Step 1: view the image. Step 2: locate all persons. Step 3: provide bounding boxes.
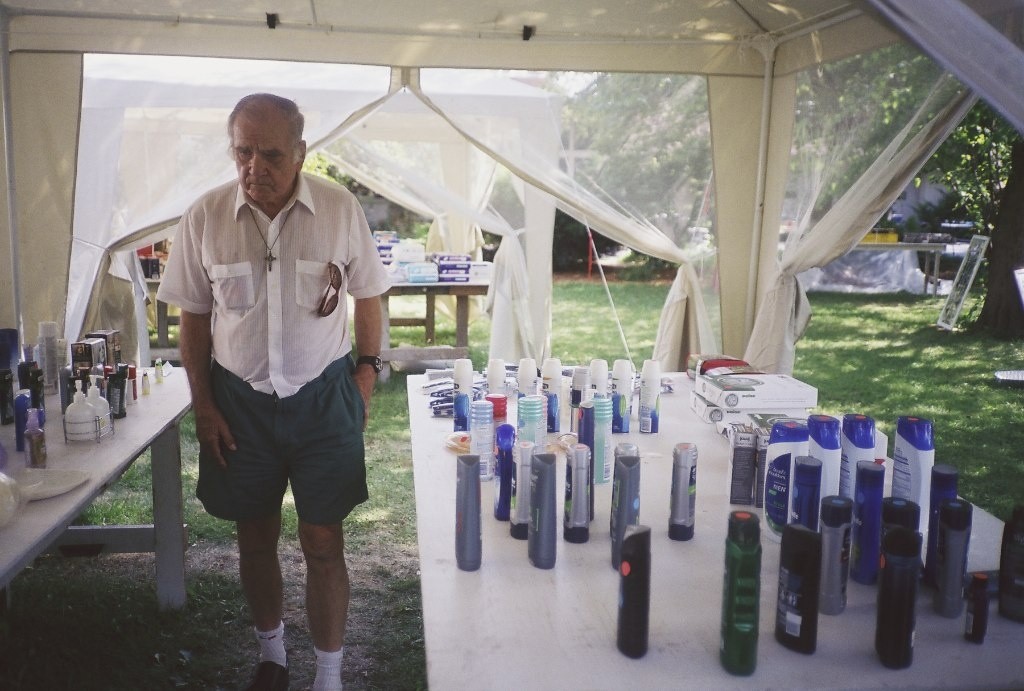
[156,93,393,690]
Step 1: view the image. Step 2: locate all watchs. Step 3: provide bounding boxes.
[357,356,383,373]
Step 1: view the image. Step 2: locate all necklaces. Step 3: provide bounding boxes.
[248,204,292,271]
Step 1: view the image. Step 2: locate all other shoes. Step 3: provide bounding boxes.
[253,655,289,691]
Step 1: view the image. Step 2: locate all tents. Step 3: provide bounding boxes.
[0,0,1024,379]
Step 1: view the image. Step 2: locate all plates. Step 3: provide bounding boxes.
[24,469,92,501]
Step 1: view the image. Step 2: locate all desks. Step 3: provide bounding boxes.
[407,373,1024,691]
[0,366,197,614]
[378,283,489,382]
[852,243,947,297]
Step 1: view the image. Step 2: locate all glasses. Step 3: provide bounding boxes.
[318,262,342,317]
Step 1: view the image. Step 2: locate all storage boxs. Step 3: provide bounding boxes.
[373,230,493,282]
[689,353,819,508]
[60,329,120,415]
